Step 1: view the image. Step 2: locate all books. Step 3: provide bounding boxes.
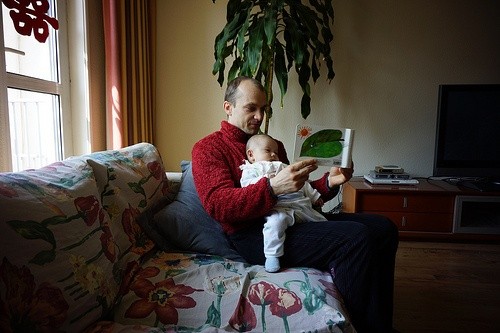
[292,123,356,168]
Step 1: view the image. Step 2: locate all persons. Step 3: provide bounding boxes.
[191,76,400,333]
[239,134,328,272]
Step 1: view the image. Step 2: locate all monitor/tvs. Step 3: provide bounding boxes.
[433,84,500,192]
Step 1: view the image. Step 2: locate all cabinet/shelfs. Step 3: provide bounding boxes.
[342,178,500,241]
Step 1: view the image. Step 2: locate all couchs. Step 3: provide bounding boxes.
[0,142,356,333]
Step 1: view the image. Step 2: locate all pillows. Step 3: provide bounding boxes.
[81,142,177,286]
[0,156,118,333]
